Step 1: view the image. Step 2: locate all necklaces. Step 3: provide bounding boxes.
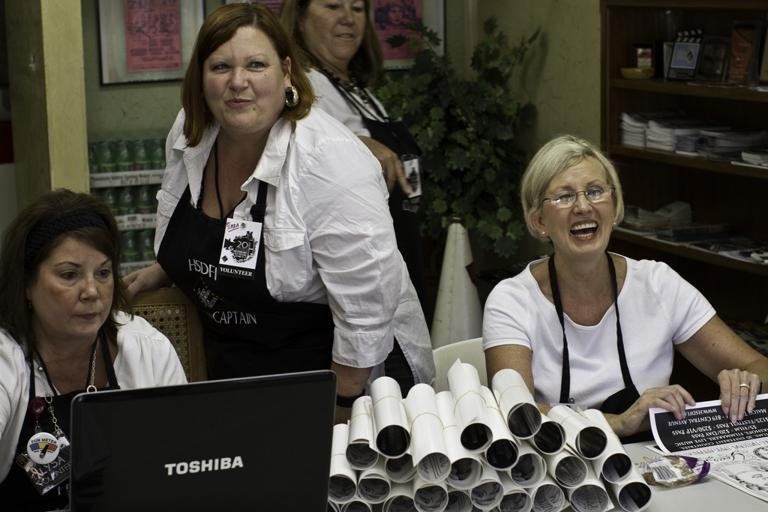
[36,341,96,431]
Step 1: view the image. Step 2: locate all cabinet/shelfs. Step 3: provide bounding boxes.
[598,0,768,277]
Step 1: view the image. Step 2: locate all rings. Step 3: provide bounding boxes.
[740,383,749,388]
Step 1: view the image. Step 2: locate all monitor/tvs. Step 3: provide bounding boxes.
[68,369,338,512]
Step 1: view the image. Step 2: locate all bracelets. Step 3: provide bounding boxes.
[336,389,366,408]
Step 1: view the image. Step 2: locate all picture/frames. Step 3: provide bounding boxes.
[223,0,283,14]
[367,0,447,70]
[96,0,206,88]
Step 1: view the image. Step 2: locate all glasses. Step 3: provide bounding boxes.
[542,183,615,209]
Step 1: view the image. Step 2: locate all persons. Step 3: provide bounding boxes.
[481,133,768,436]
[0,187,188,512]
[119,4,435,425]
[280,0,423,277]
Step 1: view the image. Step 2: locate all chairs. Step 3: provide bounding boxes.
[432,336,488,394]
[115,287,207,384]
